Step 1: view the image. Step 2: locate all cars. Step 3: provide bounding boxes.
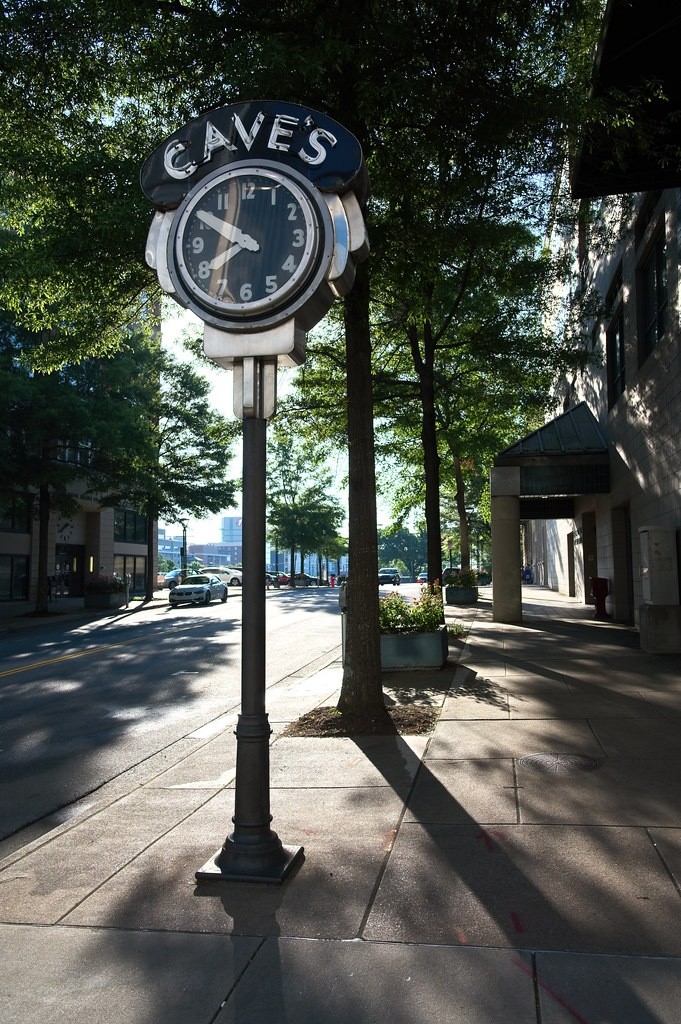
[169,575,228,607]
[441,568,460,584]
[378,568,401,586]
[200,567,242,586]
[415,574,428,583]
[266,571,319,587]
[164,569,203,591]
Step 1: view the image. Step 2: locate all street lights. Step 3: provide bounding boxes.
[179,518,189,582]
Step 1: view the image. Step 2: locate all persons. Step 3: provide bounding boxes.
[521,563,530,584]
